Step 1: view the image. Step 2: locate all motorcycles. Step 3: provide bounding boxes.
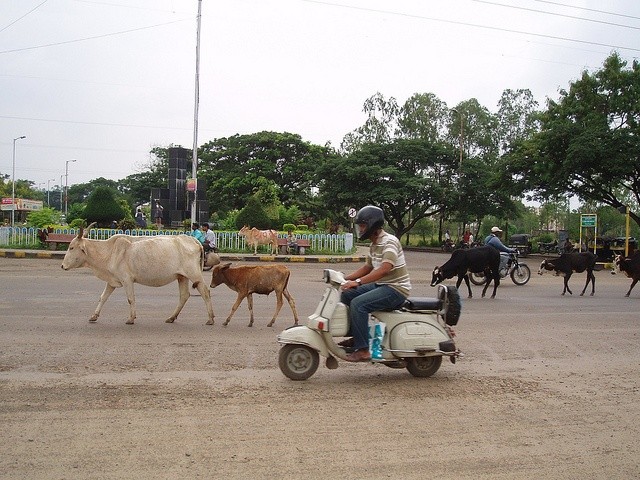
[509,233,532,257]
[469,248,531,285]
[441,238,483,253]
[200,247,219,271]
[275,269,464,381]
[537,239,562,255]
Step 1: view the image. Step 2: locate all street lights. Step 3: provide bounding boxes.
[60,174,68,214]
[47,180,55,208]
[65,160,77,218]
[12,136,26,245]
[39,182,46,193]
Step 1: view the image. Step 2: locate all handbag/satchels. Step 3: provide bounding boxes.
[204,249,220,266]
[367,313,386,359]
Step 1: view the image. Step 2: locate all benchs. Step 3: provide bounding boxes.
[45,233,76,249]
[278,239,310,254]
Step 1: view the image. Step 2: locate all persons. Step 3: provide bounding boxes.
[445,230,449,242]
[338,205,412,361]
[154,200,164,230]
[464,231,469,243]
[468,232,474,244]
[581,241,587,252]
[564,238,571,253]
[286,230,298,255]
[485,226,517,278]
[142,212,147,227]
[137,209,142,225]
[572,240,580,253]
[202,224,216,251]
[190,223,205,245]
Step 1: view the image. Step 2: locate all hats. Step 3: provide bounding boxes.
[491,226,503,233]
[193,223,200,228]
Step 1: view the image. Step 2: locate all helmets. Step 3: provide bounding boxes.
[353,205,384,242]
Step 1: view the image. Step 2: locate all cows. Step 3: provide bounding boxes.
[61,218,214,325]
[210,262,299,327]
[238,222,278,256]
[611,250,640,297]
[430,246,501,299]
[537,251,598,296]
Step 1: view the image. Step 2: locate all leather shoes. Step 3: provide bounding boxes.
[338,338,354,347]
[343,350,371,362]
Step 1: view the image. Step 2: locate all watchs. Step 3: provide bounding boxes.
[355,279,362,285]
